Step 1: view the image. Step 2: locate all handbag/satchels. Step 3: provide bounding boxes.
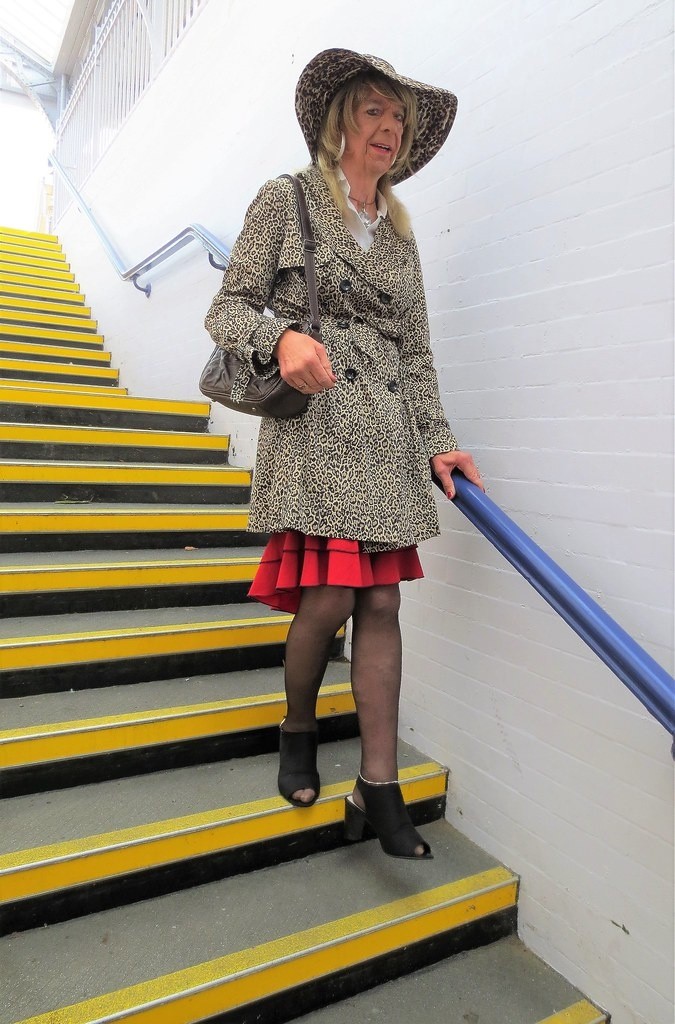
[200,324,321,420]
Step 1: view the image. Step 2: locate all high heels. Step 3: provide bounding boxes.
[342,775,437,863]
[277,718,322,808]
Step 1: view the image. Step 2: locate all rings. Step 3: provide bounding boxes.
[298,383,307,390]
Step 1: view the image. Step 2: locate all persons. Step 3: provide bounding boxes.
[202,45,484,860]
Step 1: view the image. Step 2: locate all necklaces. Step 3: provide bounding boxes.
[348,196,376,229]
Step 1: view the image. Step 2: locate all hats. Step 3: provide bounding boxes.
[297,49,458,186]
